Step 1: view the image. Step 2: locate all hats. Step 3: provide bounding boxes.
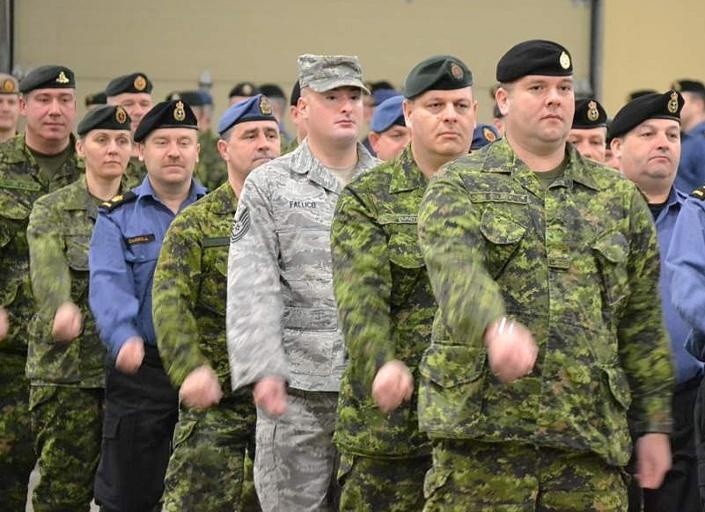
[165,82,284,105]
[366,78,408,134]
[86,72,154,104]
[289,49,371,108]
[76,104,131,135]
[569,99,606,130]
[218,94,277,135]
[133,101,198,141]
[606,89,687,142]
[17,68,75,92]
[494,39,575,84]
[467,108,504,147]
[630,78,702,100]
[403,56,475,98]
[1,73,20,95]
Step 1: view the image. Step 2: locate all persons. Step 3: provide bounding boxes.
[1,39,704,510]
